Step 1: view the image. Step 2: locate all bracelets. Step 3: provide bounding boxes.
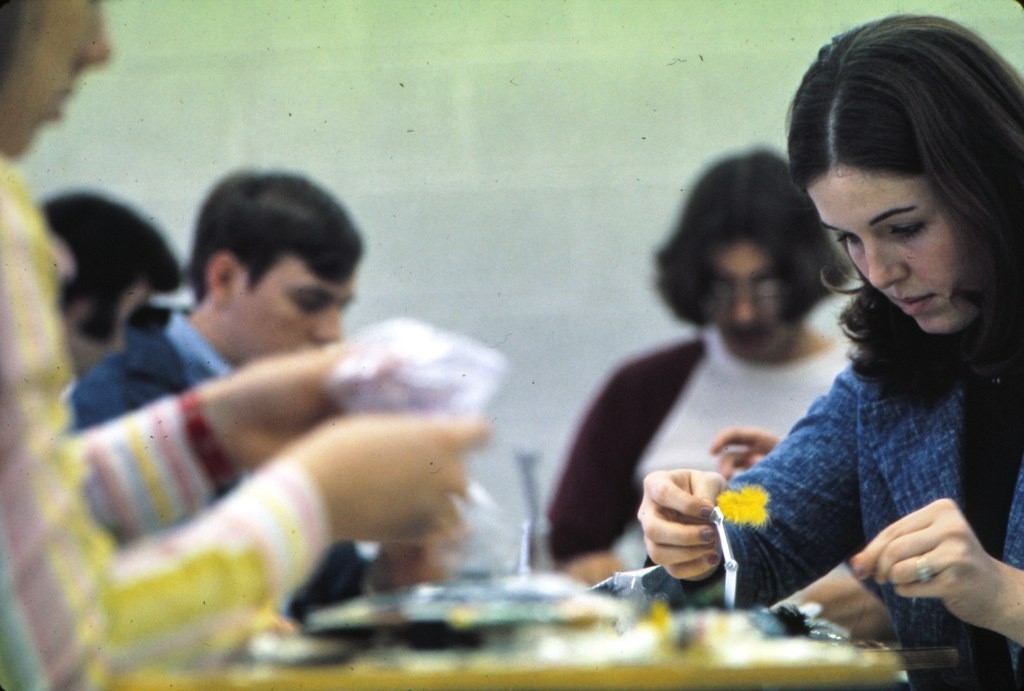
[179,389,239,492]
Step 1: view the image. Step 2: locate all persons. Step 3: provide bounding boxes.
[39,191,182,383]
[547,148,876,599]
[0,0,487,691]
[66,168,500,656]
[636,11,1024,691]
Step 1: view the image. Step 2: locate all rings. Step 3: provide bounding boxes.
[916,555,932,585]
